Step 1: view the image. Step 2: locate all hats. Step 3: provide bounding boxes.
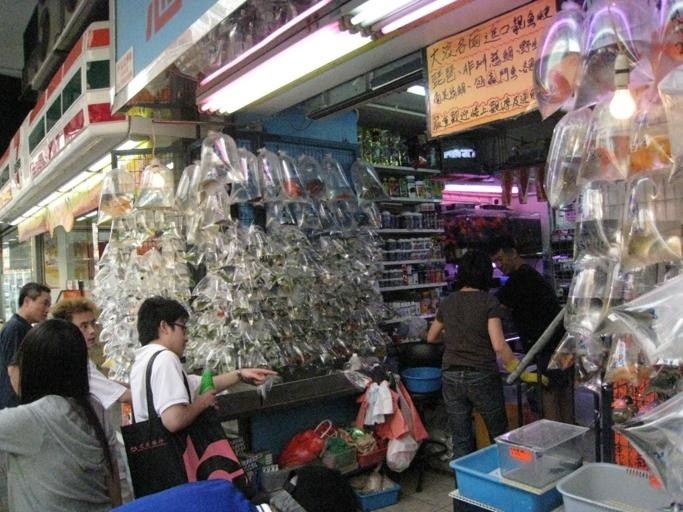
[283,467,357,511]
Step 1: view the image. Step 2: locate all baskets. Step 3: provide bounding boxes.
[322,427,400,510]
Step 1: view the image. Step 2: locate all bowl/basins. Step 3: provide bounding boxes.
[400,367,442,394]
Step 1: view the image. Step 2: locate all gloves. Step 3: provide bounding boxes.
[503,358,549,386]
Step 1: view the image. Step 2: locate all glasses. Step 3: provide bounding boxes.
[168,322,187,332]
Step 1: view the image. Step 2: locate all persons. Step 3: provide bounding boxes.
[482,233,576,425]
[107,465,360,511]
[129,295,279,435]
[48,296,131,410]
[0,319,117,512]
[0,282,52,409]
[427,251,549,493]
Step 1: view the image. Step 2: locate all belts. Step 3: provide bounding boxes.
[447,365,479,372]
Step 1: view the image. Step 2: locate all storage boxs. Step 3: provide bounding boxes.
[493,418,589,489]
[450,486,491,510]
[448,442,590,510]
[555,460,677,511]
[355,472,403,511]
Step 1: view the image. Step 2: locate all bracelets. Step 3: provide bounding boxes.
[236,368,248,384]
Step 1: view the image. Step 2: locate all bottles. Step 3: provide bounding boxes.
[199,369,215,416]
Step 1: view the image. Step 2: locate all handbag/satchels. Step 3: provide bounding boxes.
[277,419,332,465]
[120,403,250,499]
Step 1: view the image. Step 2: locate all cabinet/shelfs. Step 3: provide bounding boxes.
[355,160,447,350]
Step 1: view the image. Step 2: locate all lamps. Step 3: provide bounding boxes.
[606,2,637,120]
[194,0,486,116]
